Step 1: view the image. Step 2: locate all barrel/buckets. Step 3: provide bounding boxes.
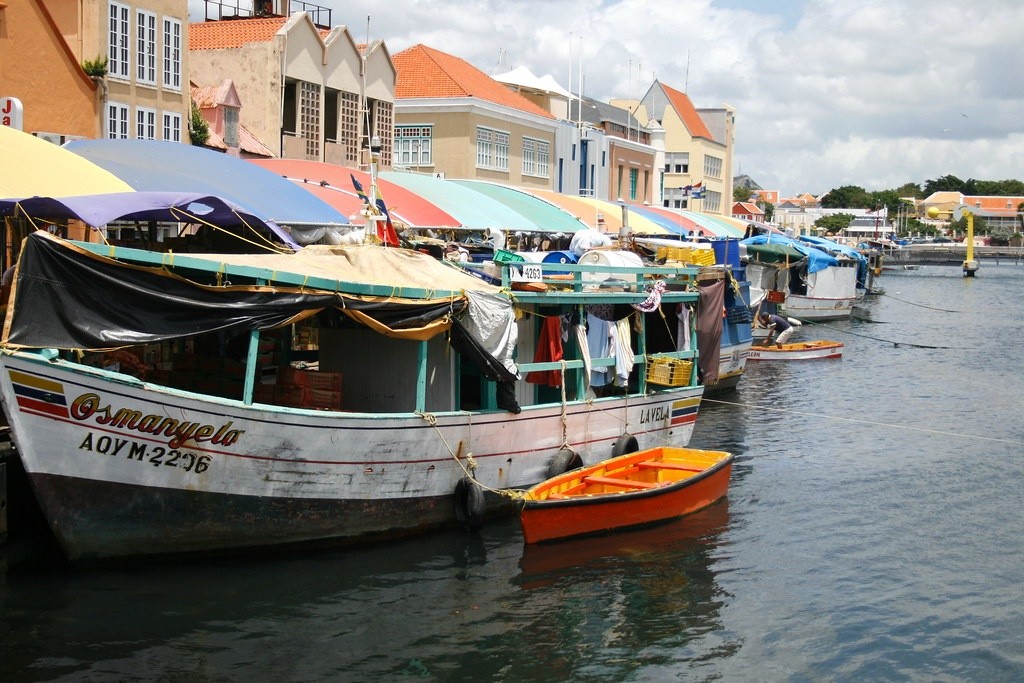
[576,249,646,292]
[542,251,581,279]
[710,239,741,282]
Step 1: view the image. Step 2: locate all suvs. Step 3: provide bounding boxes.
[911,237,927,244]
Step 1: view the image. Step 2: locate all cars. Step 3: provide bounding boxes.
[926,236,935,243]
[933,236,952,243]
[903,237,912,242]
[953,236,964,243]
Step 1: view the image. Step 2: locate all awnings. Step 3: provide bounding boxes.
[244,157,789,241]
[0,124,352,227]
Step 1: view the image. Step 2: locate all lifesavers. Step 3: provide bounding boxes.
[848,242,855,248]
[452,476,487,531]
[612,433,640,458]
[856,243,865,250]
[546,448,585,480]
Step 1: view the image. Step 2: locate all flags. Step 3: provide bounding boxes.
[679,185,692,197]
[349,172,402,248]
[692,187,706,200]
[692,181,702,188]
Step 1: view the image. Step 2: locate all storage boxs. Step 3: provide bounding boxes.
[726,305,754,324]
[274,364,342,411]
[483,249,525,279]
[657,246,716,267]
[81,336,203,394]
[645,356,693,387]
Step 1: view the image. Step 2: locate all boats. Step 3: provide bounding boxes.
[462,233,877,381]
[746,339,843,361]
[0,229,701,567]
[516,493,731,589]
[515,446,736,547]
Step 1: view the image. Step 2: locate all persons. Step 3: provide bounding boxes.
[761,312,795,349]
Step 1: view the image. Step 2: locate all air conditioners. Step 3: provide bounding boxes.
[32,131,61,147]
